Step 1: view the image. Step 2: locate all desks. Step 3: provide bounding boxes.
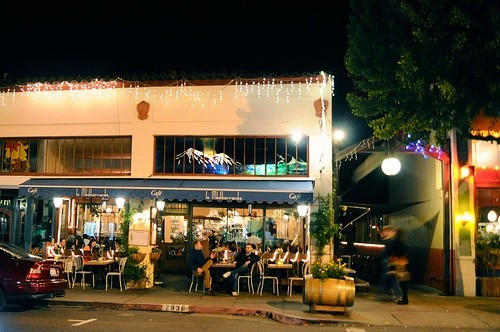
[212,261,237,293]
[268,264,292,295]
[47,254,69,258]
[83,260,115,289]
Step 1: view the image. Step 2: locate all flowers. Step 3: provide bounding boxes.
[276,248,285,255]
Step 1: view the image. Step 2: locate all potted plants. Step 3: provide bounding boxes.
[114,203,162,290]
[473,226,500,297]
[304,193,356,311]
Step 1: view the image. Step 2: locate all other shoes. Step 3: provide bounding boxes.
[223,272,230,278]
[206,289,215,295]
[398,299,409,304]
[232,292,237,296]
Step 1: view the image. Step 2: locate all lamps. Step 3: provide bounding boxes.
[156,201,165,213]
[381,152,401,175]
[487,188,497,223]
[54,198,64,209]
[297,205,307,218]
[116,198,125,208]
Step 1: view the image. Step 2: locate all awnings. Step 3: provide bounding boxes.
[18,179,314,205]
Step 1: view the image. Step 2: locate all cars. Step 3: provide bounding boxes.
[0,242,67,311]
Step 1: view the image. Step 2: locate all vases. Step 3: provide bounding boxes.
[277,255,283,265]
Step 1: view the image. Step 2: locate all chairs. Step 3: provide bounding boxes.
[47,246,127,292]
[189,249,311,298]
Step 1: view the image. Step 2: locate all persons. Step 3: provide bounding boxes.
[382,225,411,305]
[190,240,216,296]
[42,234,122,287]
[268,218,276,236]
[223,244,259,296]
[246,233,261,248]
[209,230,217,250]
[31,243,40,254]
